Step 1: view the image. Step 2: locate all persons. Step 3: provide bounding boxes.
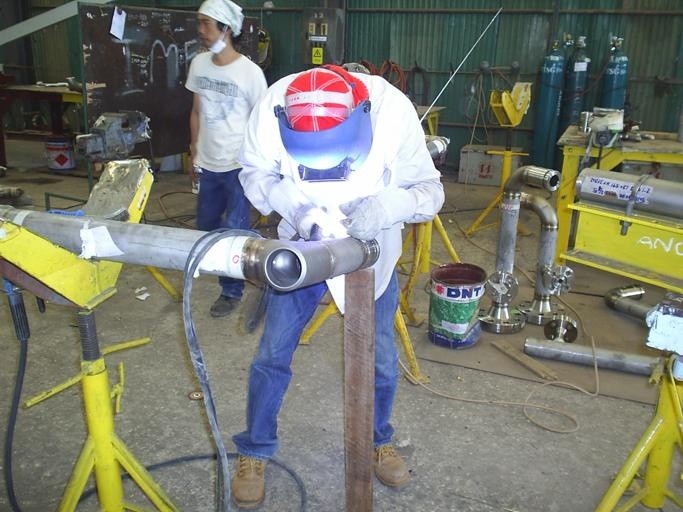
[229,64,446,508]
[184,0,267,316]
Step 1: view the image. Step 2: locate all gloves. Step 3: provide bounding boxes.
[267,175,334,241]
[338,182,417,241]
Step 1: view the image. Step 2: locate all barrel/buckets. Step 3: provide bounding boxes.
[45,139,77,170]
[427,263,490,348]
[182,152,192,173]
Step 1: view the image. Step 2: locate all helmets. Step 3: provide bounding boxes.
[274,63,373,183]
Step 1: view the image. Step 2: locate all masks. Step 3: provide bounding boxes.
[207,25,229,55]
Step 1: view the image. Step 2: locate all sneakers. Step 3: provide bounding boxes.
[209,295,242,317]
[230,454,266,508]
[373,443,411,487]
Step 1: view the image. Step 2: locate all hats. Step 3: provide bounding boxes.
[197,0,245,39]
